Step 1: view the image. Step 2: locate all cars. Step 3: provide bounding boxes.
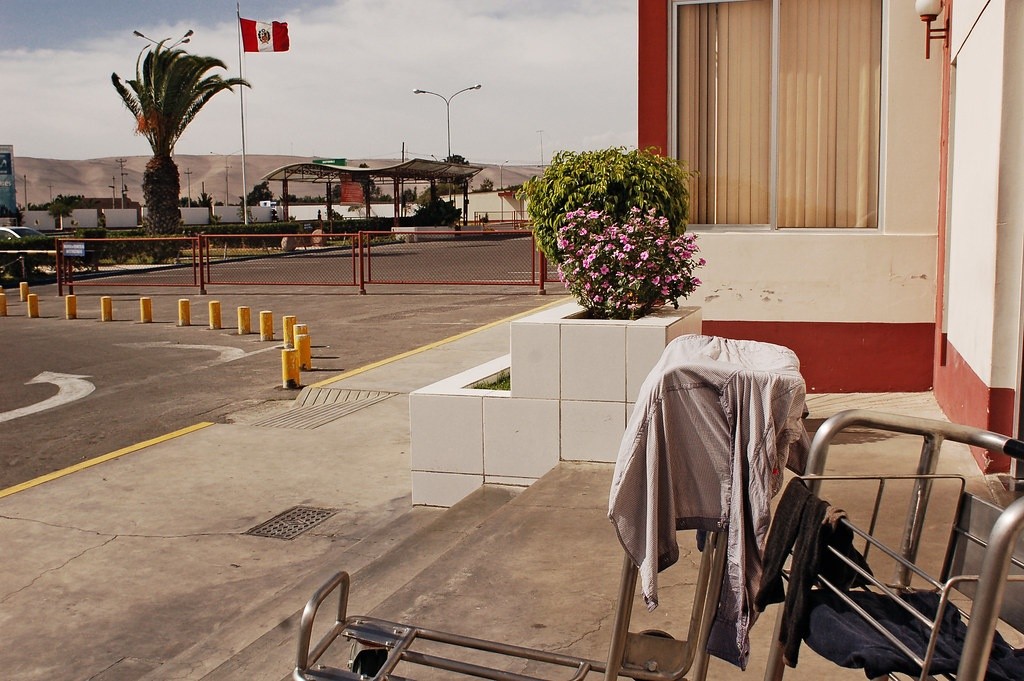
[0,226,46,241]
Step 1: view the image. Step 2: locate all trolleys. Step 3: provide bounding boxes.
[295,334,811,681]
[757,411,1024,681]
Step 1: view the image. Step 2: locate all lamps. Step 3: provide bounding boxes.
[915,0,950,60]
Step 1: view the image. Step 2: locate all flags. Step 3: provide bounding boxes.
[240,17,289,53]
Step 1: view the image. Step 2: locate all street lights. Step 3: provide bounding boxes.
[133,29,193,159]
[210,149,242,206]
[493,160,509,221]
[413,84,482,204]
